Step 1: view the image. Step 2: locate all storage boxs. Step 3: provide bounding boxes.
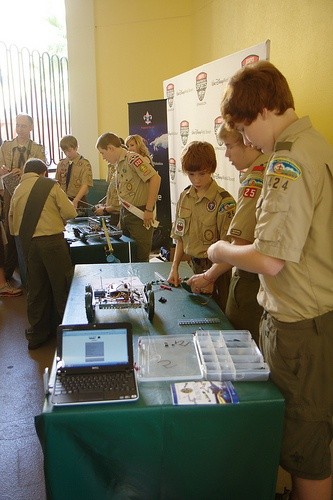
[135,328,271,383]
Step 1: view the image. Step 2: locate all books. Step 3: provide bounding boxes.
[171,379,241,406]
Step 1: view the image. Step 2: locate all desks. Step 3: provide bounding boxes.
[34,261,285,500]
[60,214,138,266]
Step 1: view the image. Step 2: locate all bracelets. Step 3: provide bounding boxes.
[102,208,109,216]
[145,207,154,212]
[203,270,215,284]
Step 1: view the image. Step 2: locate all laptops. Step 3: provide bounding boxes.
[50,323,139,406]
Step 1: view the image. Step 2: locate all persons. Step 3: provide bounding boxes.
[126,132,154,161]
[209,56,333,500]
[56,136,92,217]
[95,132,162,263]
[184,118,263,348]
[0,191,23,296]
[166,141,236,312]
[10,158,78,350]
[92,138,126,228]
[0,114,49,281]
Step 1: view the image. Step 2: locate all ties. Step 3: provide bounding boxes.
[17,147,26,168]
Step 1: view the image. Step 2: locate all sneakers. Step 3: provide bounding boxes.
[0,281,23,296]
